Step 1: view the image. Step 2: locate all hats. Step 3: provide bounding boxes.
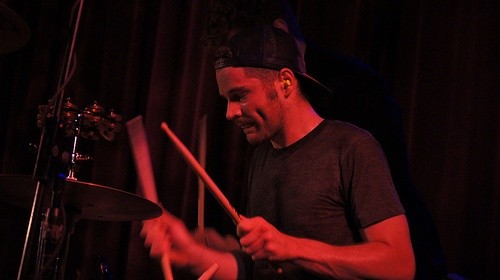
[213,23,322,88]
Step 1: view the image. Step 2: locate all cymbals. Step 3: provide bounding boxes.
[0,174,162,221]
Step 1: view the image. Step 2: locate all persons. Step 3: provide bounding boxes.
[139,24,417,280]
